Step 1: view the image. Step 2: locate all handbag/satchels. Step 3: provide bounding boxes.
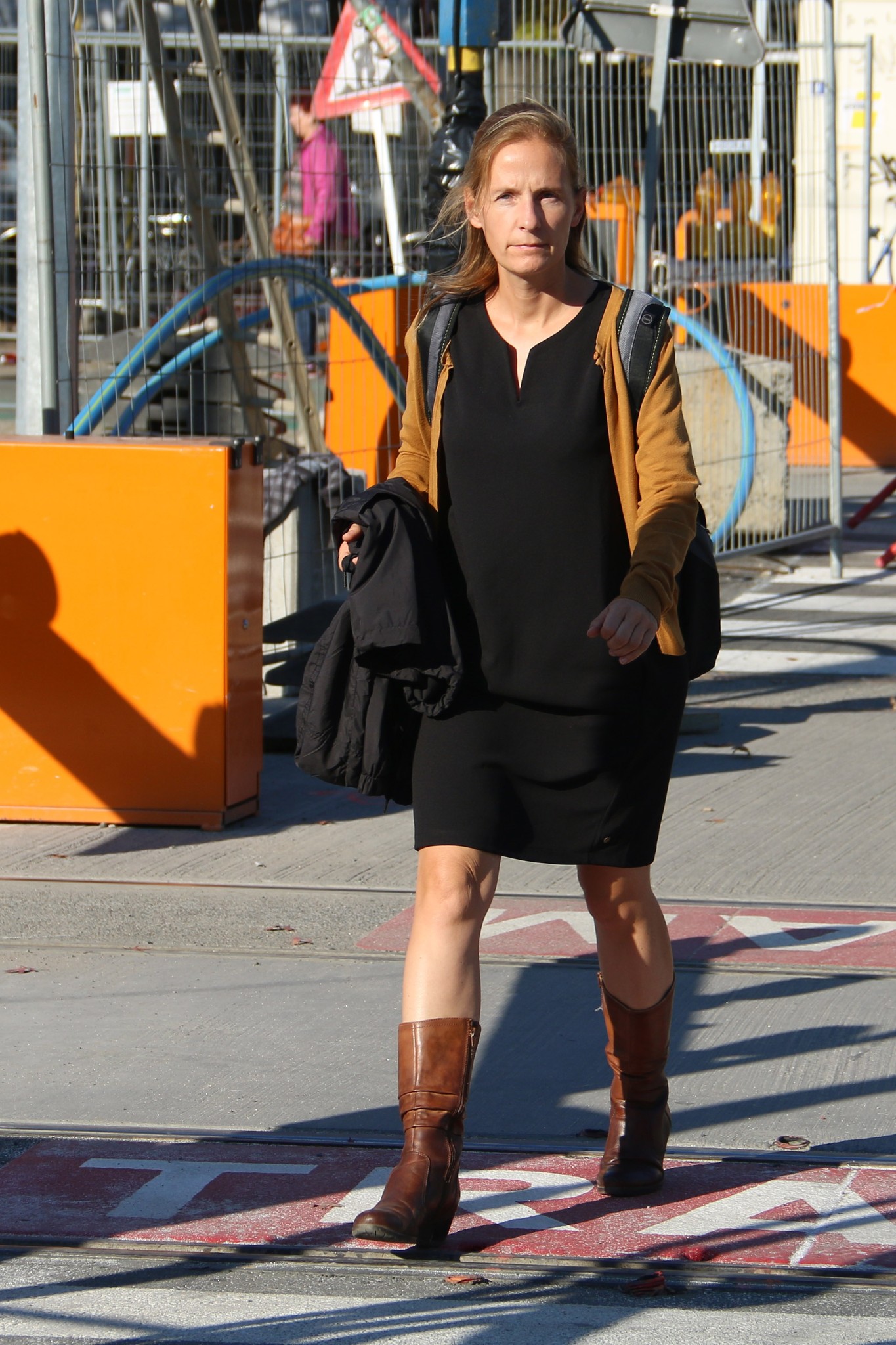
[618,290,723,679]
[272,214,316,257]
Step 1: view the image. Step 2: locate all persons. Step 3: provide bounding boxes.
[337,103,700,1242]
[277,95,357,374]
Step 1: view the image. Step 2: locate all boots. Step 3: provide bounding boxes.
[352,1017,482,1244]
[594,971,676,1196]
